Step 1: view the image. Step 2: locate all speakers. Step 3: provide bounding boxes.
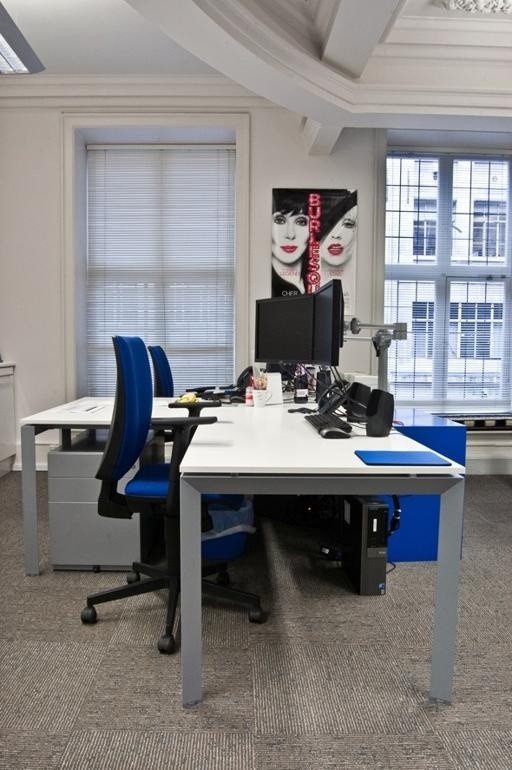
[238,366,253,387]
[365,388,395,437]
[346,382,371,423]
[316,371,331,401]
[295,375,308,403]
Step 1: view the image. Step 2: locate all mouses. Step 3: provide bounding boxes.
[320,426,351,439]
[230,395,245,403]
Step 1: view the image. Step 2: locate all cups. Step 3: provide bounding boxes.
[252,389,271,407]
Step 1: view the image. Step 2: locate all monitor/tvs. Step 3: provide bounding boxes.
[312,279,344,367]
[255,293,314,364]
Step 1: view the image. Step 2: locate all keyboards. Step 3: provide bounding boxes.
[305,412,352,433]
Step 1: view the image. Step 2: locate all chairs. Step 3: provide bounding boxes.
[81,339,268,656]
[148,344,221,444]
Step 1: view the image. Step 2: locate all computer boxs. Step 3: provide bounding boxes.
[339,495,389,597]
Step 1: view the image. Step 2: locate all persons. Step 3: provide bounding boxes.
[272,189,307,296]
[314,189,358,291]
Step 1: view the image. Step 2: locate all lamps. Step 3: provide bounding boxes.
[0,4,45,75]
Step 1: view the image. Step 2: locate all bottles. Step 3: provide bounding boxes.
[294,370,308,404]
[245,386,255,408]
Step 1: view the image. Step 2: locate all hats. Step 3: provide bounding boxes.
[316,189,357,241]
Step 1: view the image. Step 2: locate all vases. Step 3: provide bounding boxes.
[253,390,269,407]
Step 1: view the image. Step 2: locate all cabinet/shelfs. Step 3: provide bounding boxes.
[47,430,166,570]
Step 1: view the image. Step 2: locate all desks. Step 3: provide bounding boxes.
[23,394,467,706]
[387,407,468,564]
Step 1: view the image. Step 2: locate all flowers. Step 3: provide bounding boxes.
[250,374,270,390]
